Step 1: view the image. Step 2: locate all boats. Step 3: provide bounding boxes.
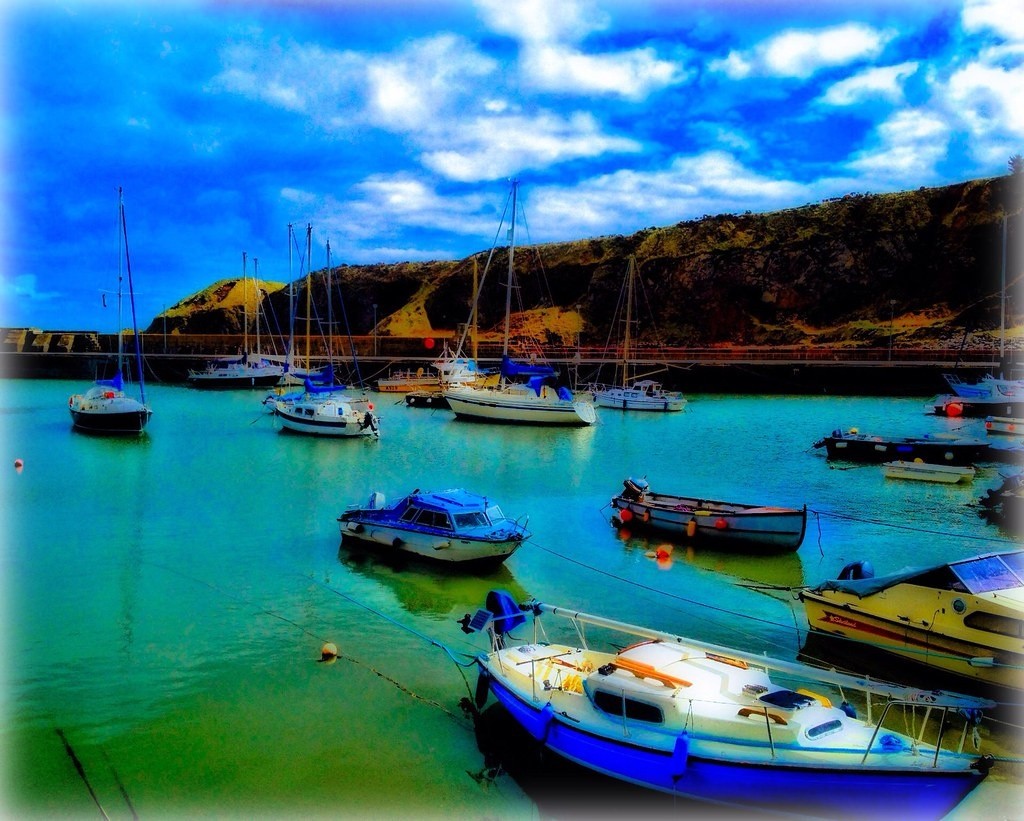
[977,473,1023,518]
[616,531,804,608]
[923,393,1023,417]
[796,548,1023,735]
[336,488,534,574]
[611,477,807,555]
[813,428,993,466]
[984,415,1024,436]
[405,386,451,409]
[457,590,999,821]
[881,460,975,484]
[337,548,535,621]
[376,368,442,392]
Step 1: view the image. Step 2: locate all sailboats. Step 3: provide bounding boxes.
[435,177,691,426]
[186,220,382,439]
[67,186,154,438]
[942,207,1023,398]
